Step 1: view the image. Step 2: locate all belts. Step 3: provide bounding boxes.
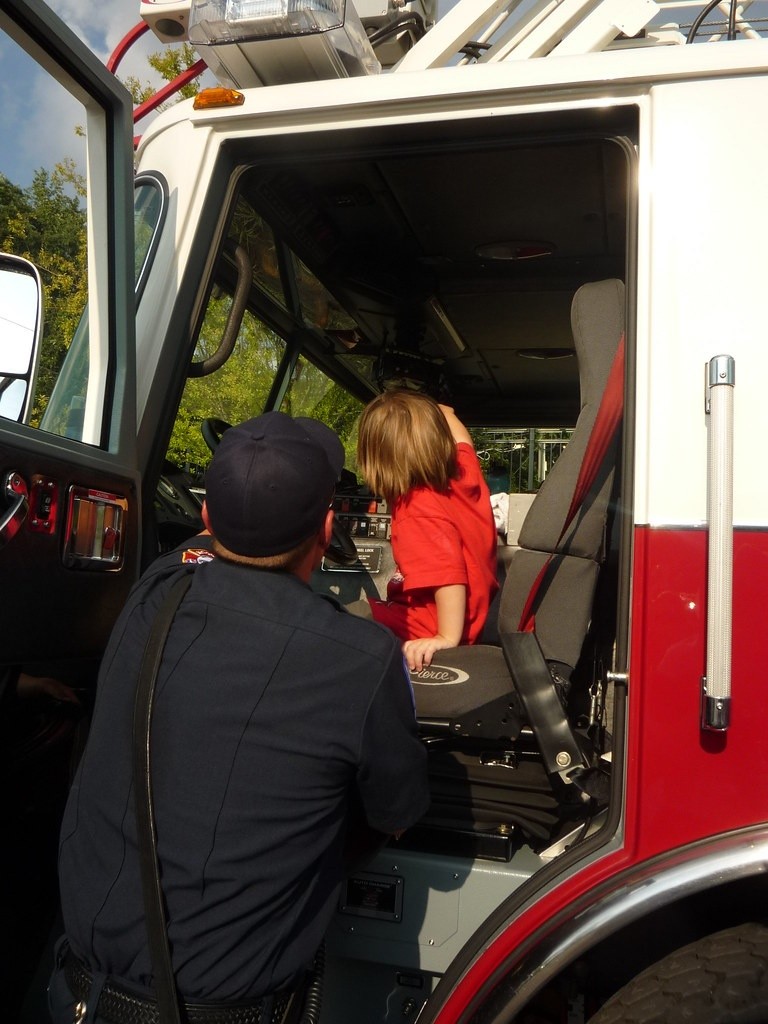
[65,960,288,1024]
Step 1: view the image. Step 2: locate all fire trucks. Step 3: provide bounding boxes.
[0,0,767,1022]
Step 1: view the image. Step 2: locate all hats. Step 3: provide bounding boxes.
[206,411,345,558]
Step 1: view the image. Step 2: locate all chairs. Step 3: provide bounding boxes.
[401,278,628,725]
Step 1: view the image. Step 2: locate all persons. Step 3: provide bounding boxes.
[356,389,498,673]
[44,410,431,1024]
